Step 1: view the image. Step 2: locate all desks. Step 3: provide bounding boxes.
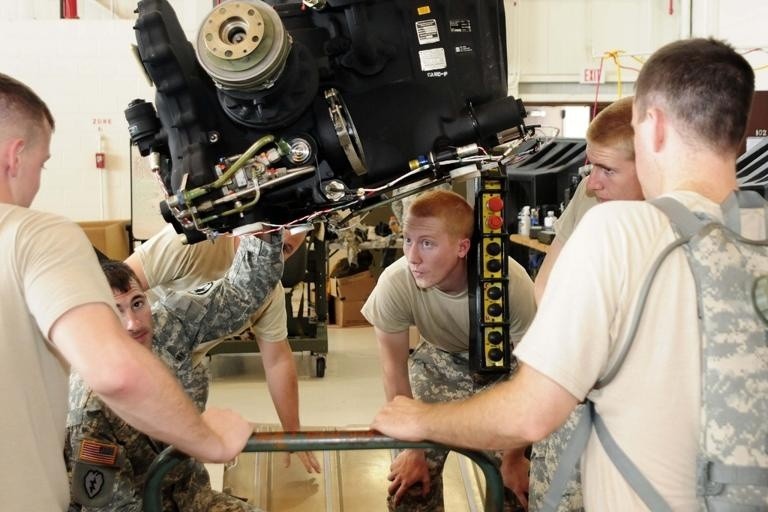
[508,233,551,264]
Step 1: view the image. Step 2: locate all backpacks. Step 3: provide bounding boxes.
[542,189,764,510]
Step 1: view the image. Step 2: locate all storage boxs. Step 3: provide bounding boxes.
[329,273,378,301]
[74,218,133,267]
[333,297,376,331]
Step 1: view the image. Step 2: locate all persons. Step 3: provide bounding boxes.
[389,179,454,228]
[119,216,322,474]
[58,224,286,511]
[357,189,540,511]
[370,35,768,512]
[528,94,647,512]
[0,71,257,511]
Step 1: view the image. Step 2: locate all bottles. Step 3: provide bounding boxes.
[544,211,557,232]
[388,216,399,234]
[517,205,539,237]
